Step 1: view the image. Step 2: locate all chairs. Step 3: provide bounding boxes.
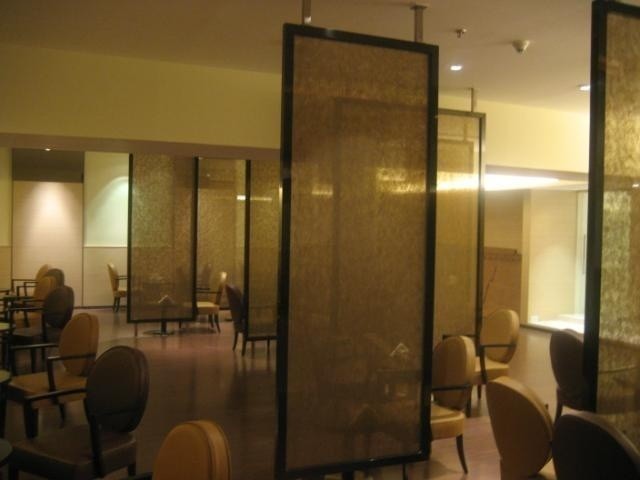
[550,329,639,480]
[107,263,127,313]
[178,263,228,335]
[442,310,520,418]
[486,376,555,480]
[134,323,137,337]
[365,335,476,480]
[8,345,150,480]
[1,263,100,480]
[224,283,276,356]
[118,419,232,480]
